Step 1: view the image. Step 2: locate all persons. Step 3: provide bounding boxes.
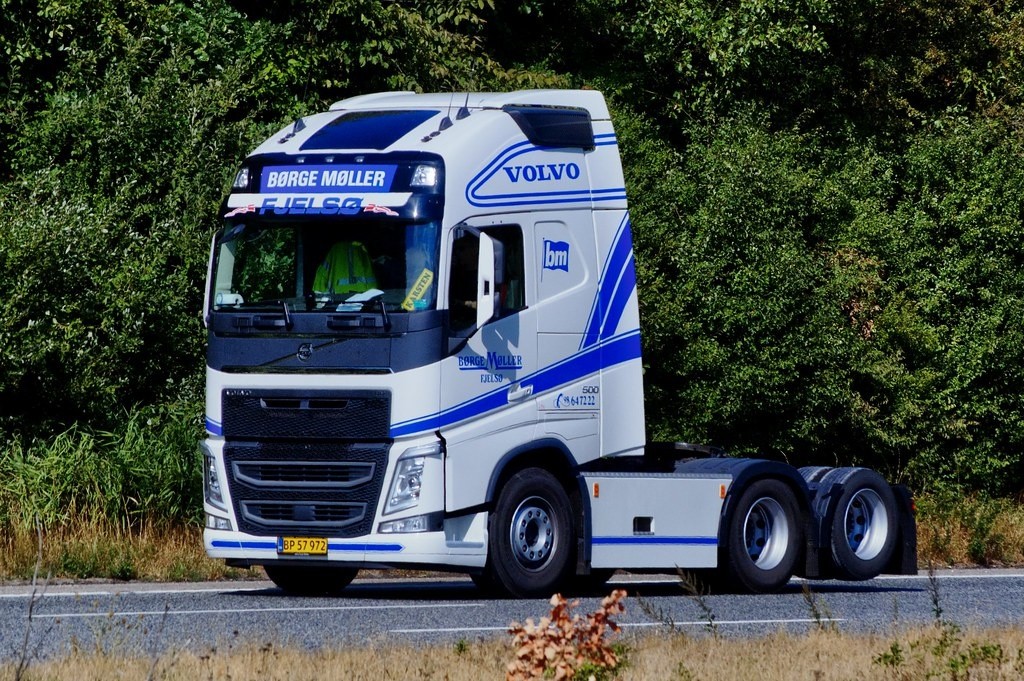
[451,235,509,332]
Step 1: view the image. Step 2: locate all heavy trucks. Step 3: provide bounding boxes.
[197,86,921,598]
